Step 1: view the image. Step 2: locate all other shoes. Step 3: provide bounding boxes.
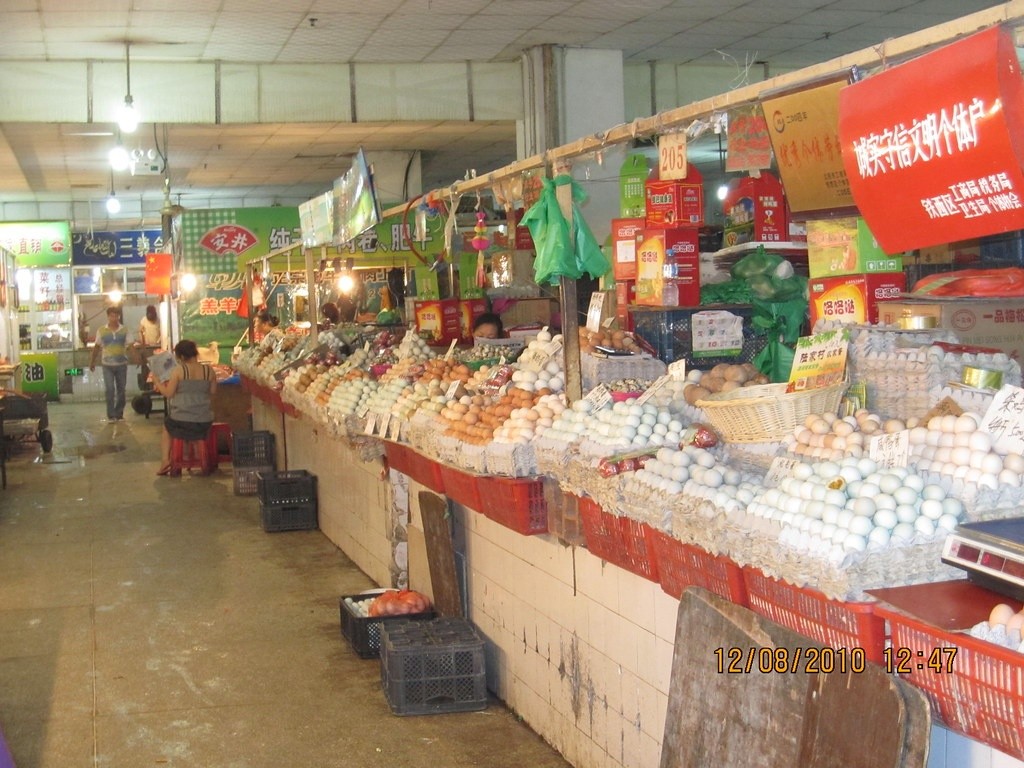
[117,416,124,422]
[108,417,115,423]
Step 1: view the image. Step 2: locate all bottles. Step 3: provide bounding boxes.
[17,298,72,312]
[663,249,679,306]
[20,320,73,351]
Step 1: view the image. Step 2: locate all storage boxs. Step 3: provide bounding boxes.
[233,465,278,497]
[5,395,48,431]
[231,429,276,468]
[581,155,1024,386]
[255,470,321,529]
[3,417,40,437]
[403,206,551,341]
[338,591,489,717]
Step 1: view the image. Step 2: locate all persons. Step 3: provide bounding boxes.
[471,312,503,349]
[257,313,283,351]
[92,307,135,424]
[140,305,162,392]
[151,340,217,476]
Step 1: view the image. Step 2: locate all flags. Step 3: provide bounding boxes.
[145,254,172,295]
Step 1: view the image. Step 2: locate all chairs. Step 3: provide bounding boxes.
[139,353,168,420]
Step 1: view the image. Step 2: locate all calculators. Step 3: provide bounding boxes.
[595,344,635,355]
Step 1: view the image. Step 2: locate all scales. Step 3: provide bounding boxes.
[939,515,1024,604]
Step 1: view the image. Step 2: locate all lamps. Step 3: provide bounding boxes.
[104,41,140,217]
[109,281,123,305]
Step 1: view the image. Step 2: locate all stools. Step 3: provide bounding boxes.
[169,419,235,481]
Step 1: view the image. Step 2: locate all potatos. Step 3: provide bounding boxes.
[682,362,769,406]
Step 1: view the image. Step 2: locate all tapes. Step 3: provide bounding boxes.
[960,365,1002,390]
[897,315,937,328]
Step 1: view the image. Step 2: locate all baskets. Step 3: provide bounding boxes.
[694,381,852,442]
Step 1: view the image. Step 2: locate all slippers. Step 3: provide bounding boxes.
[157,464,172,475]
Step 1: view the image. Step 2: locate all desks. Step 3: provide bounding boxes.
[0,362,23,394]
[209,383,252,454]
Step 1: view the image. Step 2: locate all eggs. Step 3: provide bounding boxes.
[927,345,943,361]
[345,590,430,617]
[258,330,688,464]
[989,604,1024,641]
[631,409,1024,551]
